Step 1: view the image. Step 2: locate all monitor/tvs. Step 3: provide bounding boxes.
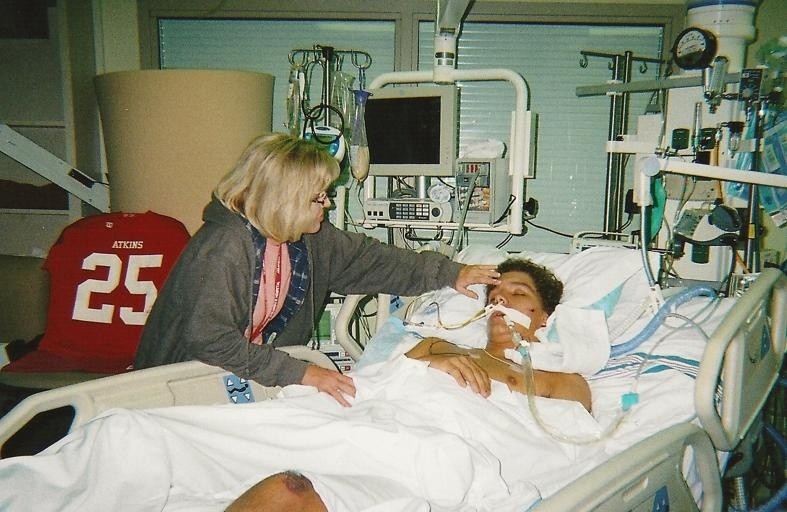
[362,89,460,178]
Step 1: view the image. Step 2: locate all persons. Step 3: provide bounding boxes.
[219,257,591,511]
[131,135,501,409]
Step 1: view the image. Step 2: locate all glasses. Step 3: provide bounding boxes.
[308,190,338,208]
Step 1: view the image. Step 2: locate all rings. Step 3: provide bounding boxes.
[335,388,341,391]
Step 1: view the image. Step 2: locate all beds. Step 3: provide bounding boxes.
[0,267,787,512]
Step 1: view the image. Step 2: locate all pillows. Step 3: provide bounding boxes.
[407,243,659,346]
